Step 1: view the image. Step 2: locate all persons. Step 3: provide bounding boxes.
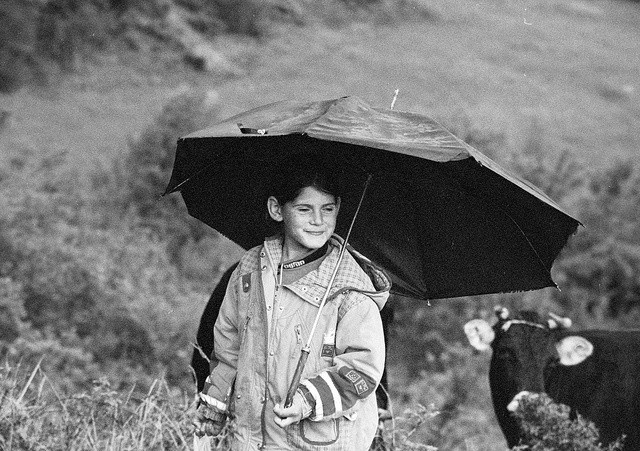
[191,157,392,450]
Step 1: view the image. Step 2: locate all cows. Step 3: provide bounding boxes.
[462,304,640,451]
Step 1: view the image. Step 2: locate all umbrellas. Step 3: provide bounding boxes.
[162,86,585,419]
